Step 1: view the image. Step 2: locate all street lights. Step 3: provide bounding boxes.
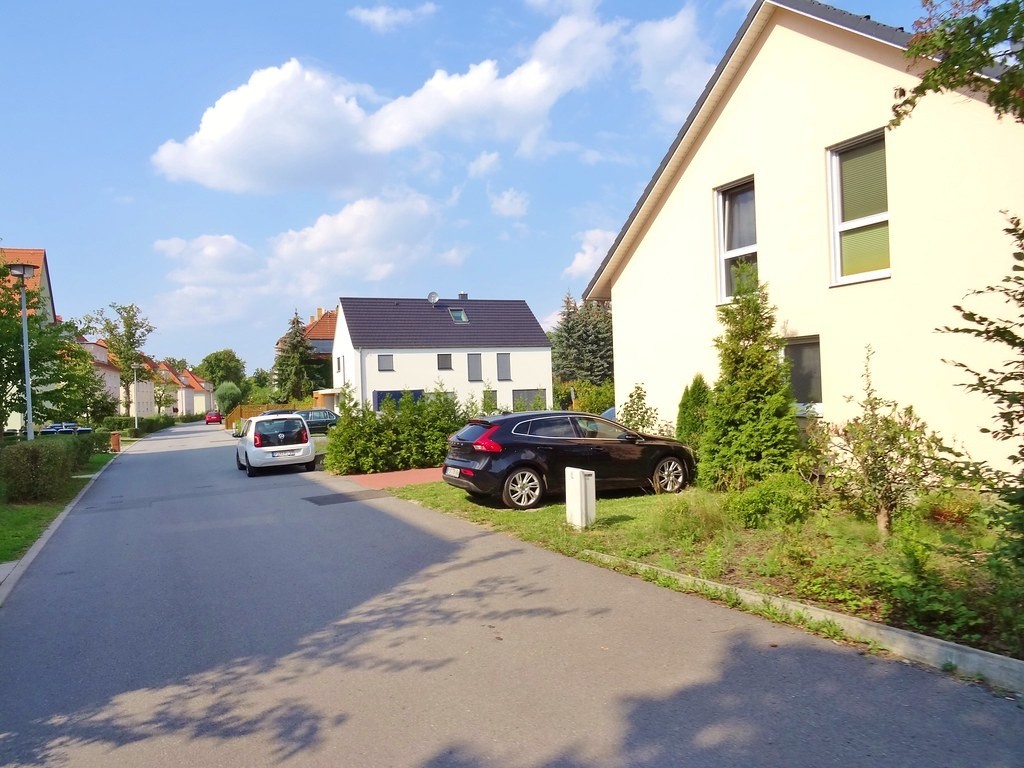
[4,262,40,443]
[130,362,141,428]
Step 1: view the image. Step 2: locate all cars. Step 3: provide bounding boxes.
[231,413,316,477]
[442,409,697,511]
[205,412,224,424]
[258,408,299,431]
[293,409,341,435]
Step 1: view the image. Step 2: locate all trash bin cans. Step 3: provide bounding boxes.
[110,431,122,452]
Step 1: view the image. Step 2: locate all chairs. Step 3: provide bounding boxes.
[257,423,270,434]
[284,421,297,431]
